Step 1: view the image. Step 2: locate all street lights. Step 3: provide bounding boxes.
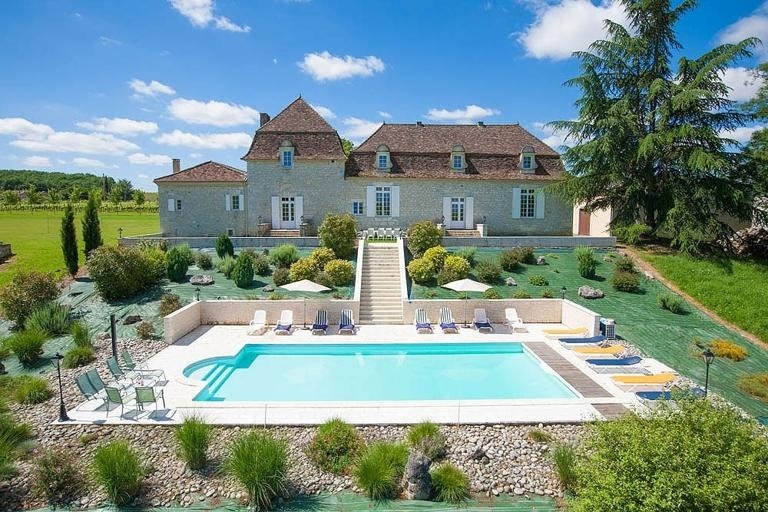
[195,287,201,300]
[561,286,566,298]
[49,351,70,422]
[701,348,714,400]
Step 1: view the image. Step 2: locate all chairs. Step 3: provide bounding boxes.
[73,351,167,420]
[415,307,526,334]
[246,309,354,335]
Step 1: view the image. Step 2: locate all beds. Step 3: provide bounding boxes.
[542,327,705,403]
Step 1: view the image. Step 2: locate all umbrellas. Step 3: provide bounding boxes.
[441,278,494,321]
[278,278,332,323]
